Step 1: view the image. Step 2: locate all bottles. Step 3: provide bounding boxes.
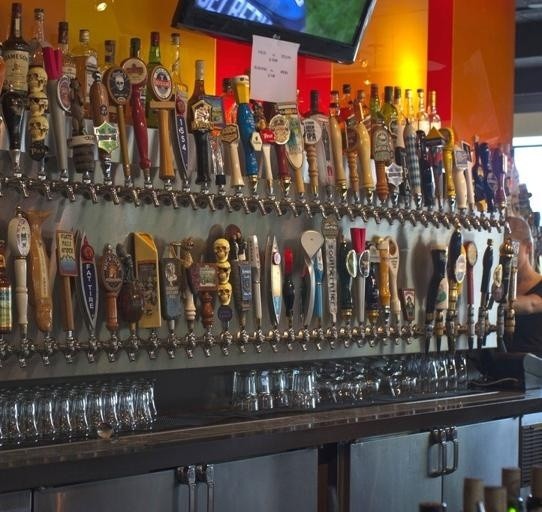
[0,0,442,168]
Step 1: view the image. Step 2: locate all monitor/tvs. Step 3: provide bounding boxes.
[171,0,377,65]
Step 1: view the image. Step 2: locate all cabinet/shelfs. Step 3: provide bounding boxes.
[349,419,523,512]
[33,446,320,512]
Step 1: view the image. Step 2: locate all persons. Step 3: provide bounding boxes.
[497,214,542,361]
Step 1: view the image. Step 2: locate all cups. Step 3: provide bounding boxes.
[231,353,469,414]
[0,377,158,448]
